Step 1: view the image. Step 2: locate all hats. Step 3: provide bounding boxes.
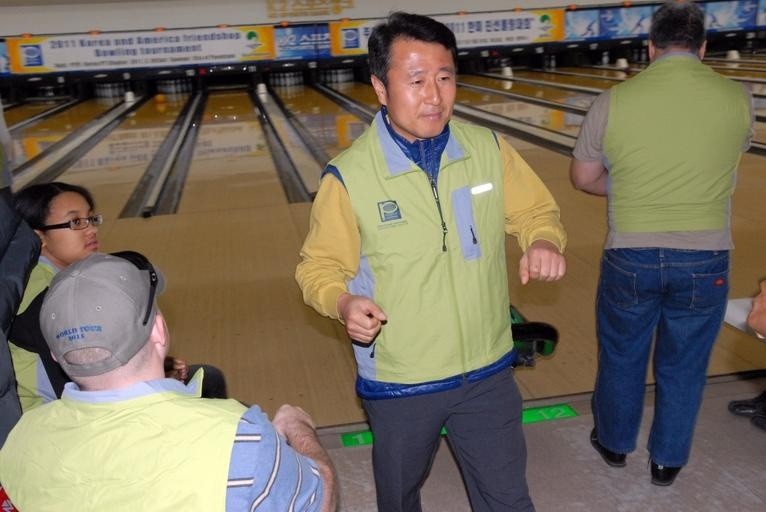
[38,251,165,378]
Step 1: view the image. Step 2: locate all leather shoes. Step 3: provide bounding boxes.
[751,413,766,430]
[650,456,681,486]
[590,427,626,466]
[729,395,765,416]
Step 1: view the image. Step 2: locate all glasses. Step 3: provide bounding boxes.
[37,213,102,230]
[109,251,158,326]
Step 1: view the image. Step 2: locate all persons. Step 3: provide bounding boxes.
[294,11,568,512]
[0,251,338,512]
[570,1,756,486]
[8,183,227,414]
[728,278,766,431]
